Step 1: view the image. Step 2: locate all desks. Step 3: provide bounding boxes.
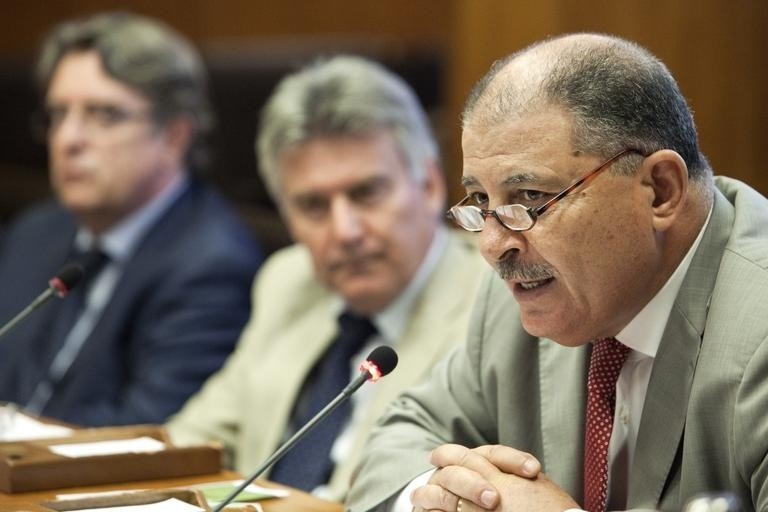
[1,412,342,512]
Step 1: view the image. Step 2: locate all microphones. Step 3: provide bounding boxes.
[212,345,399,512]
[0,263,83,337]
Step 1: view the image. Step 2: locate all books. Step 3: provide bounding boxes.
[0,424,224,496]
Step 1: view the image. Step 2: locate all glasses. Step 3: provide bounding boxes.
[448,148,649,232]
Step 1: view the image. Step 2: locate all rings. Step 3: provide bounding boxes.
[455,496,463,512]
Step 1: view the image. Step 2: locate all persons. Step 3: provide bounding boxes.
[154,50,500,512]
[338,29,768,512]
[0,9,264,431]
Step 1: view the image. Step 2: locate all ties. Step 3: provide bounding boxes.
[583,336,633,512]
[12,245,110,403]
[264,313,379,493]
